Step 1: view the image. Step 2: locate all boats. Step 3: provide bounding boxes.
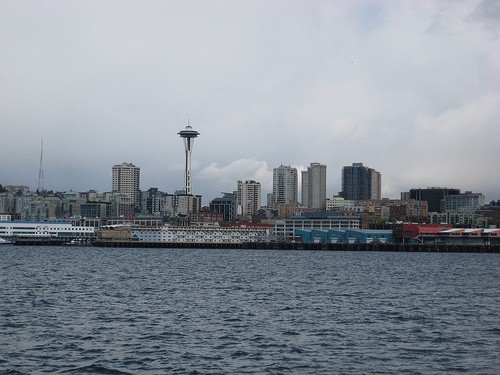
[0,219,97,245]
